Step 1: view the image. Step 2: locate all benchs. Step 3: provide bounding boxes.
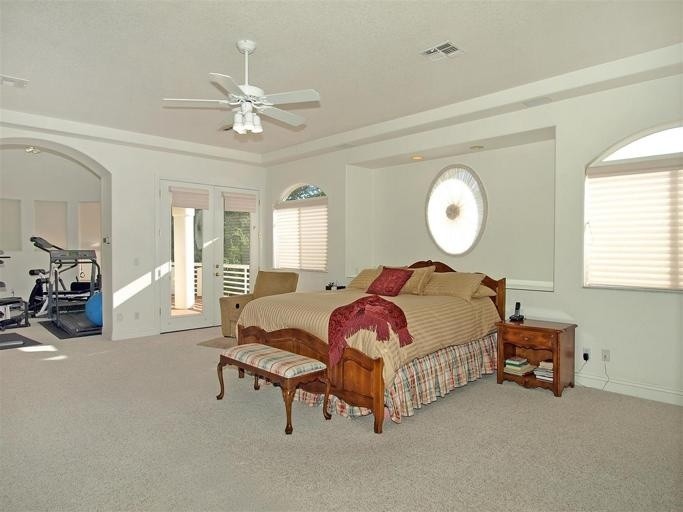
[216,342,332,434]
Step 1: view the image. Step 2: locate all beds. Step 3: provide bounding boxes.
[235,260,506,433]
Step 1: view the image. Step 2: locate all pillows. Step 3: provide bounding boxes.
[366,267,415,297]
[364,265,436,295]
[472,283,498,299]
[424,272,487,303]
[347,269,377,290]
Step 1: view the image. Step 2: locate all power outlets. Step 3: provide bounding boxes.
[602,349,610,362]
[583,347,592,359]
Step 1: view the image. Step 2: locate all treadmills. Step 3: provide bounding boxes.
[49,250,102,337]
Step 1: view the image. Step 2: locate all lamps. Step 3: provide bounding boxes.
[25,145,40,154]
[232,101,263,134]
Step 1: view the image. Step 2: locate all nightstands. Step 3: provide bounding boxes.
[495,319,577,397]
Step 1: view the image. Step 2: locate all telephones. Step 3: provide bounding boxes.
[509,302,524,321]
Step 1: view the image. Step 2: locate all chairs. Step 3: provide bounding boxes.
[219,271,299,338]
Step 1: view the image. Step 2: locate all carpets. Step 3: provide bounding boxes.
[197,337,238,349]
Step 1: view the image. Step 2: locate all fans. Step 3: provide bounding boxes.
[162,40,321,128]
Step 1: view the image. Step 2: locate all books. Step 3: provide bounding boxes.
[501,355,553,384]
